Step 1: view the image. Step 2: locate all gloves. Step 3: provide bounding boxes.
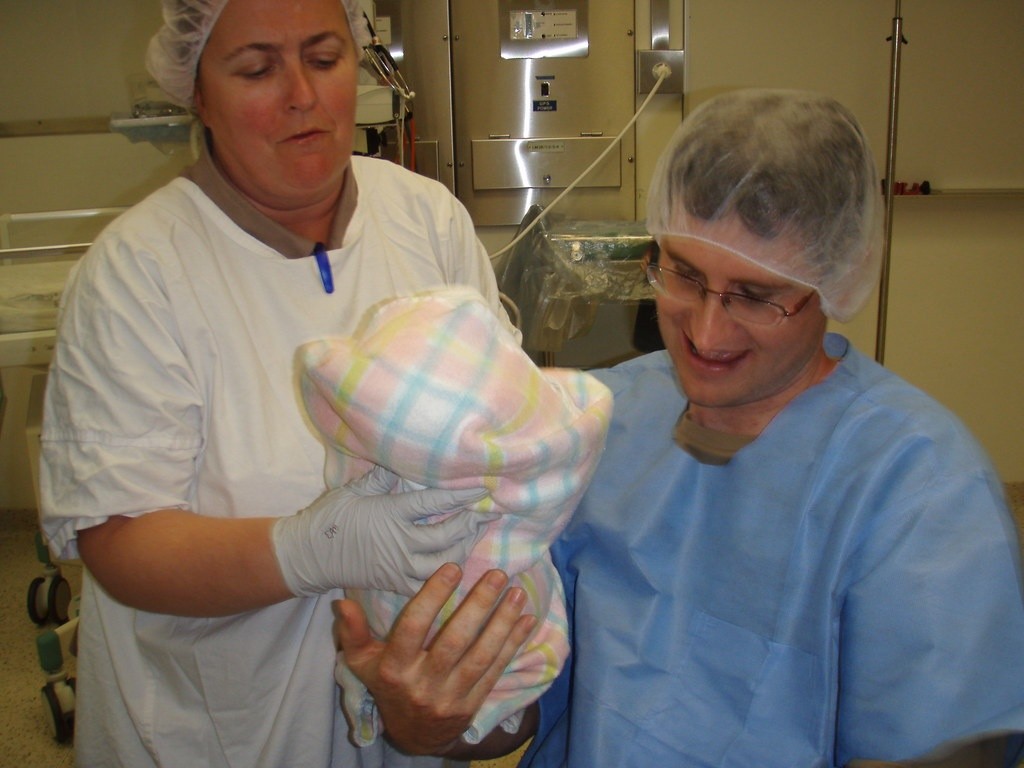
[272,465,505,602]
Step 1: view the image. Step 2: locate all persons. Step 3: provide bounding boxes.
[294,283,612,749]
[32,1,521,768]
[331,90,1024,768]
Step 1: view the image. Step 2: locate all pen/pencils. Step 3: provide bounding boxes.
[308,241,339,294]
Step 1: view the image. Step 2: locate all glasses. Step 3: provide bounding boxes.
[645,262,819,327]
